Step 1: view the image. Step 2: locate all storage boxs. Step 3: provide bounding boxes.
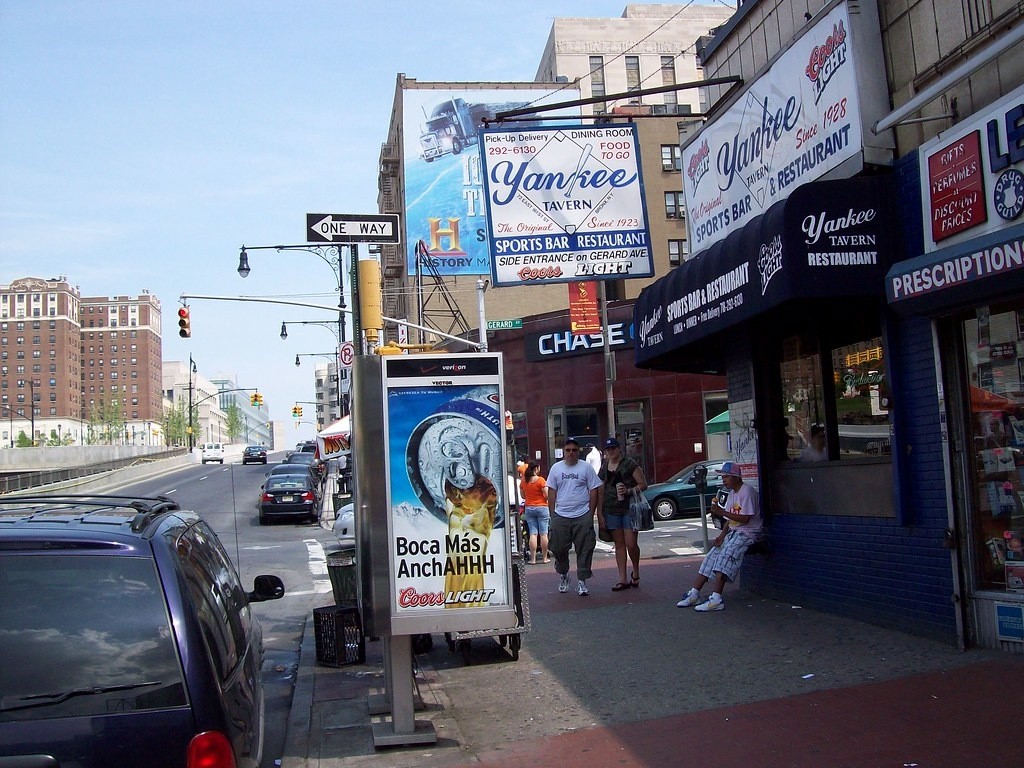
[314,603,366,668]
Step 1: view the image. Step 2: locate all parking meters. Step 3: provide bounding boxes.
[693,464,711,555]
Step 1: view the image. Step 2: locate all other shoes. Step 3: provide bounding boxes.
[543,559,551,563]
[527,560,536,565]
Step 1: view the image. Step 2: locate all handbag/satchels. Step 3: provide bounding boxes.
[629,487,655,532]
[598,519,614,542]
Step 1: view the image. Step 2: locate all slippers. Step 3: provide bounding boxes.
[612,582,631,591]
[631,571,640,587]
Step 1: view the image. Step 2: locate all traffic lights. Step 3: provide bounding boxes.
[250,393,263,406]
[291,405,302,417]
[177,306,191,337]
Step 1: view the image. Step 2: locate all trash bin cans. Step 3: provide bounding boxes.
[332,490,353,519]
[327,547,359,627]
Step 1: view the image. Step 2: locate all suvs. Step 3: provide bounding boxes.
[1,495,285,768]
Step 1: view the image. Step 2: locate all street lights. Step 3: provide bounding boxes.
[106,416,180,446]
[5,377,34,448]
[58,424,61,447]
[188,352,197,453]
[280,320,350,417]
[87,424,90,446]
[236,242,364,358]
[205,416,249,444]
[294,353,344,418]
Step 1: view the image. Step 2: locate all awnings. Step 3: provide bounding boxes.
[705,410,731,435]
[632,180,905,379]
[314,414,351,462]
[884,221,1024,320]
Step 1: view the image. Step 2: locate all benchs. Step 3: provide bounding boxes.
[274,483,303,488]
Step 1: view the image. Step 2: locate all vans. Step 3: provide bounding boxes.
[200,442,224,464]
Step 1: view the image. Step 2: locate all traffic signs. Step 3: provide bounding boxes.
[305,212,400,247]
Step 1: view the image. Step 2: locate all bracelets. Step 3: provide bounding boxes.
[720,509,725,515]
[628,489,631,496]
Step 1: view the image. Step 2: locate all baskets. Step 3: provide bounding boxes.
[313,606,366,666]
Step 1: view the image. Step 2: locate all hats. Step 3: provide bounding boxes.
[565,437,579,445]
[583,443,595,449]
[714,462,742,477]
[811,425,826,437]
[604,438,621,449]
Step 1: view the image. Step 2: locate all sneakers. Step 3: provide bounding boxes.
[577,579,590,596]
[694,595,725,611]
[677,591,701,607]
[559,573,572,593]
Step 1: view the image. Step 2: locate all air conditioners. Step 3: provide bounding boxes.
[681,211,685,219]
[663,164,674,171]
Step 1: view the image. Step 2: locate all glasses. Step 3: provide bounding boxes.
[564,447,578,453]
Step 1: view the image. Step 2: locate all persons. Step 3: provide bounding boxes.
[987,419,1007,449]
[676,462,763,611]
[597,438,647,591]
[800,425,828,461]
[996,543,1006,560]
[519,461,551,565]
[548,439,597,596]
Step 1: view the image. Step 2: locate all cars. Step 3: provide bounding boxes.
[282,452,314,465]
[639,458,735,521]
[296,439,317,453]
[242,445,268,465]
[264,464,322,497]
[977,447,1024,518]
[259,473,322,524]
